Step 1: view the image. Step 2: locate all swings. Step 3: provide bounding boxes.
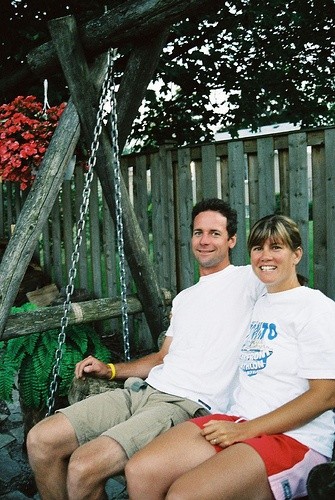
[44,47,335,500]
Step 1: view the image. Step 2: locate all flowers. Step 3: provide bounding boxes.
[0,94,68,191]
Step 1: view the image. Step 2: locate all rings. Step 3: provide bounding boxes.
[213,439,217,444]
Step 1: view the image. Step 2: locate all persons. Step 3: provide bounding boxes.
[124,215,335,500]
[27,197,267,500]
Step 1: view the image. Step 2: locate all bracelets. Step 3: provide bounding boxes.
[107,363,116,380]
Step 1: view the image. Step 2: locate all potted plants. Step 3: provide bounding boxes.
[0,302,112,451]
